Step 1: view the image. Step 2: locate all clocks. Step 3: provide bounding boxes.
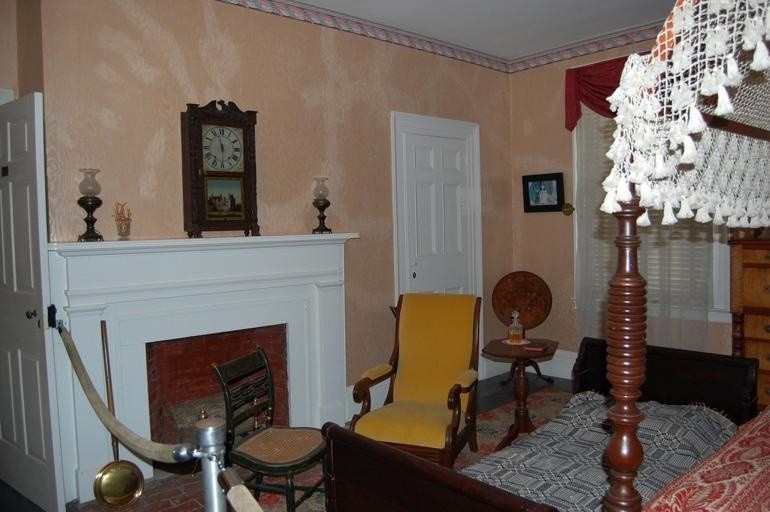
[180,100,260,239]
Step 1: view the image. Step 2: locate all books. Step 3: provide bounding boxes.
[523,342,550,351]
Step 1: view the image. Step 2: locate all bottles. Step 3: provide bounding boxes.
[507,310,525,344]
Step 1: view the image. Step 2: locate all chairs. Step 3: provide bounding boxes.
[210,345,331,512]
[351,293,482,468]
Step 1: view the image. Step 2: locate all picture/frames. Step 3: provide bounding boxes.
[522,171,565,213]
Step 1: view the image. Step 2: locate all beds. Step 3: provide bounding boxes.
[621,408,770,512]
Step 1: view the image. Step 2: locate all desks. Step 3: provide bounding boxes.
[480,330,558,450]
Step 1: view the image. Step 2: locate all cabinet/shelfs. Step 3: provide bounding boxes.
[721,228,770,410]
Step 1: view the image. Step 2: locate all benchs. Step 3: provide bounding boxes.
[318,334,761,512]
